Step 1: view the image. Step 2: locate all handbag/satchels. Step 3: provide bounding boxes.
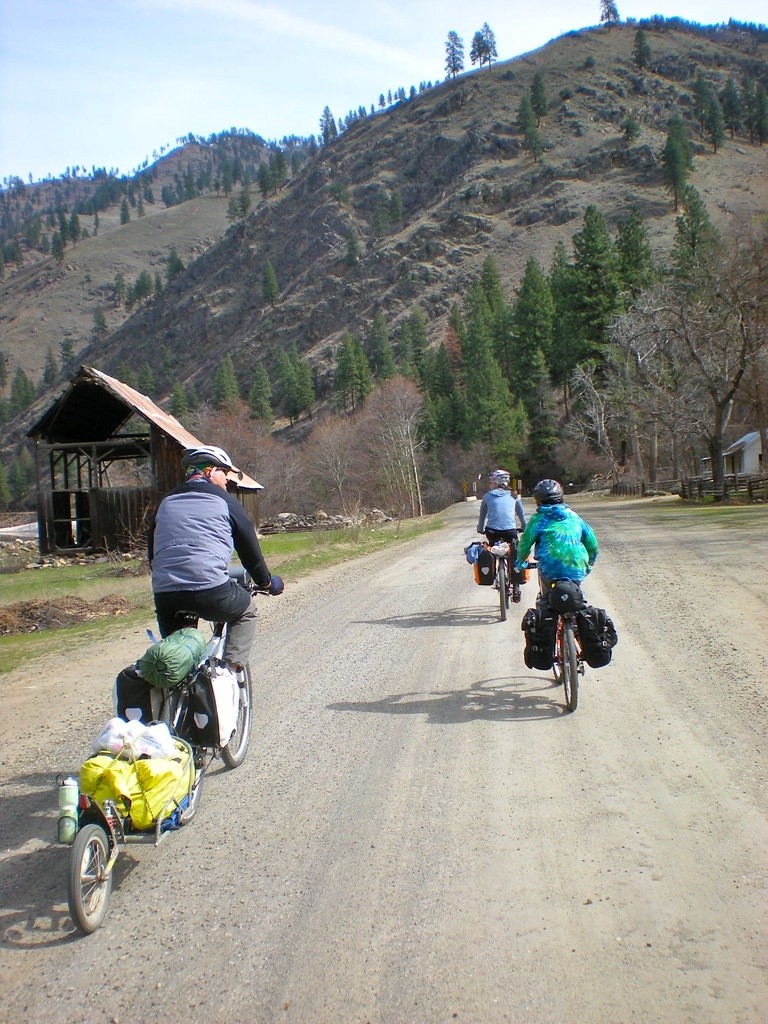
[576,606,617,668]
[474,544,496,585]
[112,663,170,748]
[510,540,531,584]
[188,657,239,749]
[521,608,558,670]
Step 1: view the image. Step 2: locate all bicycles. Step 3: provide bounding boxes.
[528,562,585,713]
[484,528,520,621]
[155,566,272,829]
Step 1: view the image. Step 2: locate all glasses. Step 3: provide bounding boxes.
[222,466,229,475]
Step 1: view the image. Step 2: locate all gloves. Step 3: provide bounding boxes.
[515,559,528,571]
[270,575,284,596]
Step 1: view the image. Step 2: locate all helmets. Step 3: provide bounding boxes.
[181,445,232,470]
[488,470,510,485]
[534,479,563,504]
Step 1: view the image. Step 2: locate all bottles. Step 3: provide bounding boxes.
[58,776,78,842]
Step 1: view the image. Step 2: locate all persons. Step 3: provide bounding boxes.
[147,446,284,675]
[476,470,527,602]
[515,479,598,663]
